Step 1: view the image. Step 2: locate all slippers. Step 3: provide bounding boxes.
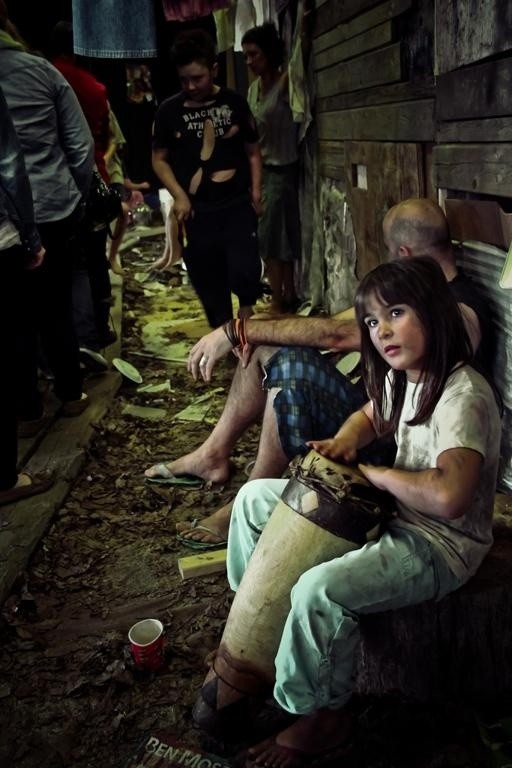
[146,462,204,485]
[176,517,227,549]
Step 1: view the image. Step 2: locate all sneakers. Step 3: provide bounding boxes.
[1,464,57,502]
[78,346,108,371]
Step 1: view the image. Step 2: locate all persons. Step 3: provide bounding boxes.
[226,257,501,768]
[2,0,149,505]
[144,198,494,548]
[147,21,298,331]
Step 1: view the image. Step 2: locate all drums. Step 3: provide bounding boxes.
[192,448,401,746]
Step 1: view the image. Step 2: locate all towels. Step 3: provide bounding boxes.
[71,1,159,60]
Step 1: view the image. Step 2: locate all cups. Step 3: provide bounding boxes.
[128,619,166,675]
